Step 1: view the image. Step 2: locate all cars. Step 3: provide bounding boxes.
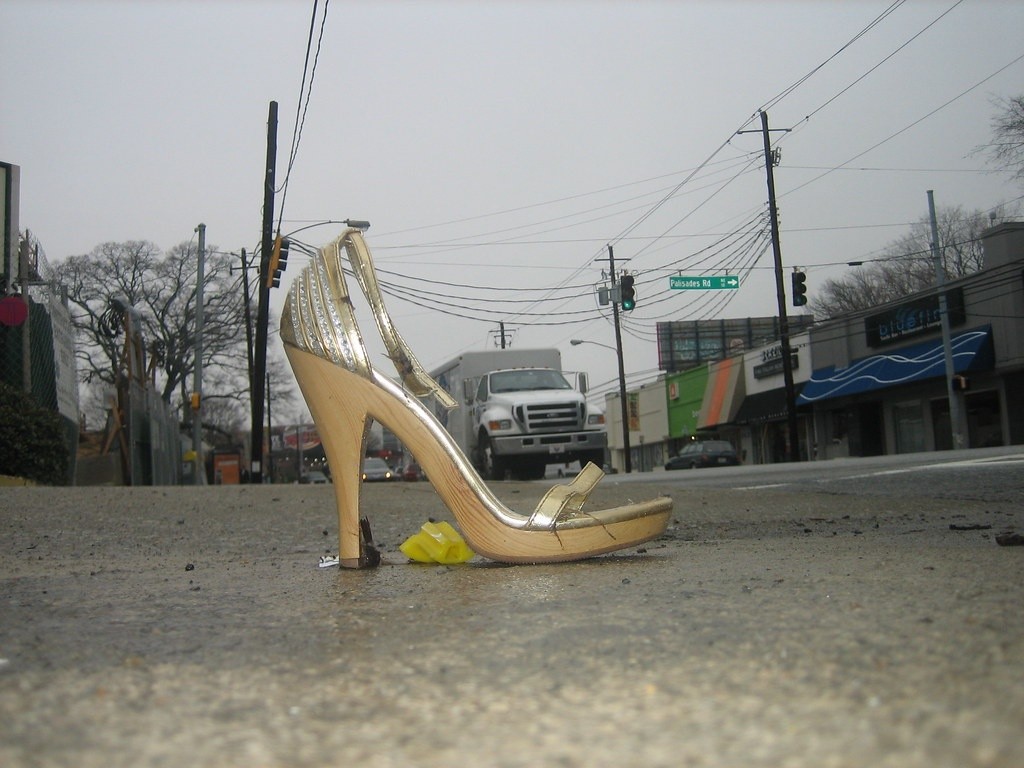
[396,463,420,483]
[363,457,398,482]
[665,439,741,471]
[303,466,333,484]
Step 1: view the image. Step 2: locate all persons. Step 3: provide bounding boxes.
[216,469,222,484]
[240,465,249,483]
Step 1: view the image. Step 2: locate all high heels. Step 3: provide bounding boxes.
[278,226,674,570]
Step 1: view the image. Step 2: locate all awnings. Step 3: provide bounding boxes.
[734,325,997,426]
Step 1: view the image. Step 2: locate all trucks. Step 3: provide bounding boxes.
[410,348,610,483]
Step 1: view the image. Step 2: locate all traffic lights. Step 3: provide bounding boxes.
[791,272,809,307]
[192,393,203,412]
[954,375,967,391]
[269,233,290,290]
[620,275,637,312]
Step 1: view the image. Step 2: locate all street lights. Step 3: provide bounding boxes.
[569,337,632,474]
[248,219,372,483]
